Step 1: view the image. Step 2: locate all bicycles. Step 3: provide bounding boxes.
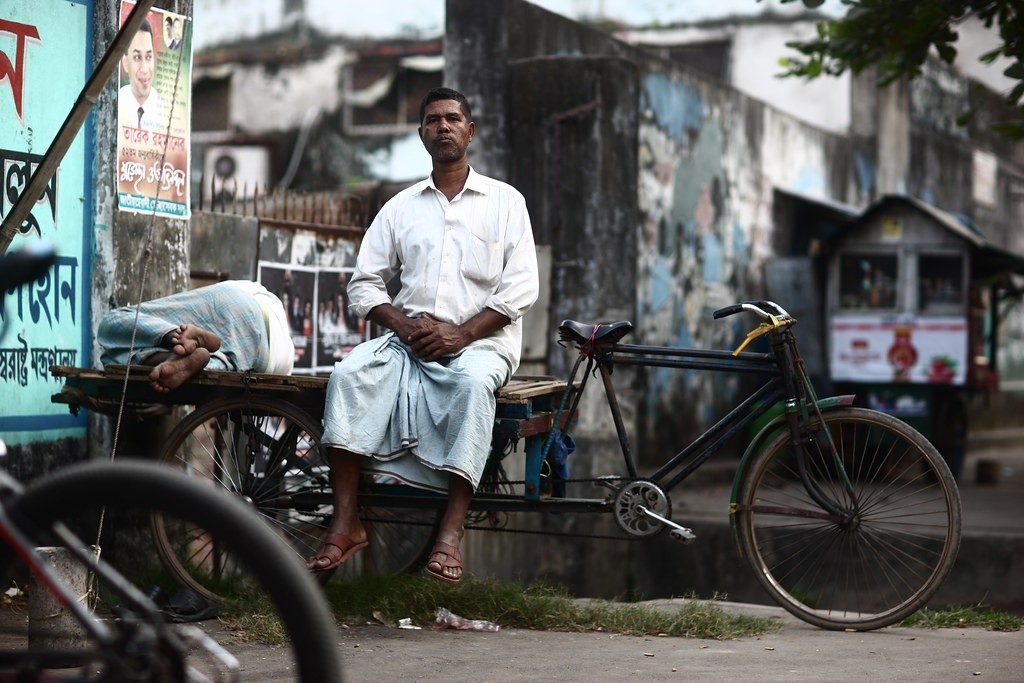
[1,240,348,681]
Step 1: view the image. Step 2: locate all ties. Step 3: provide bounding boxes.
[138,107,144,129]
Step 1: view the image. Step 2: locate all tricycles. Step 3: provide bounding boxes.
[151,286,964,635]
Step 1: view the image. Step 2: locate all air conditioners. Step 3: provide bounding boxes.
[204,144,270,200]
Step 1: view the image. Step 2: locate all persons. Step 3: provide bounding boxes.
[172,18,183,50]
[119,17,162,134]
[296,87,542,584]
[164,17,174,48]
[98,278,295,392]
[251,223,373,369]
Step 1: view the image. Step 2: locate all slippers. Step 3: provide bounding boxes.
[110,585,171,616]
[160,586,222,621]
[308,531,370,571]
[424,541,462,584]
